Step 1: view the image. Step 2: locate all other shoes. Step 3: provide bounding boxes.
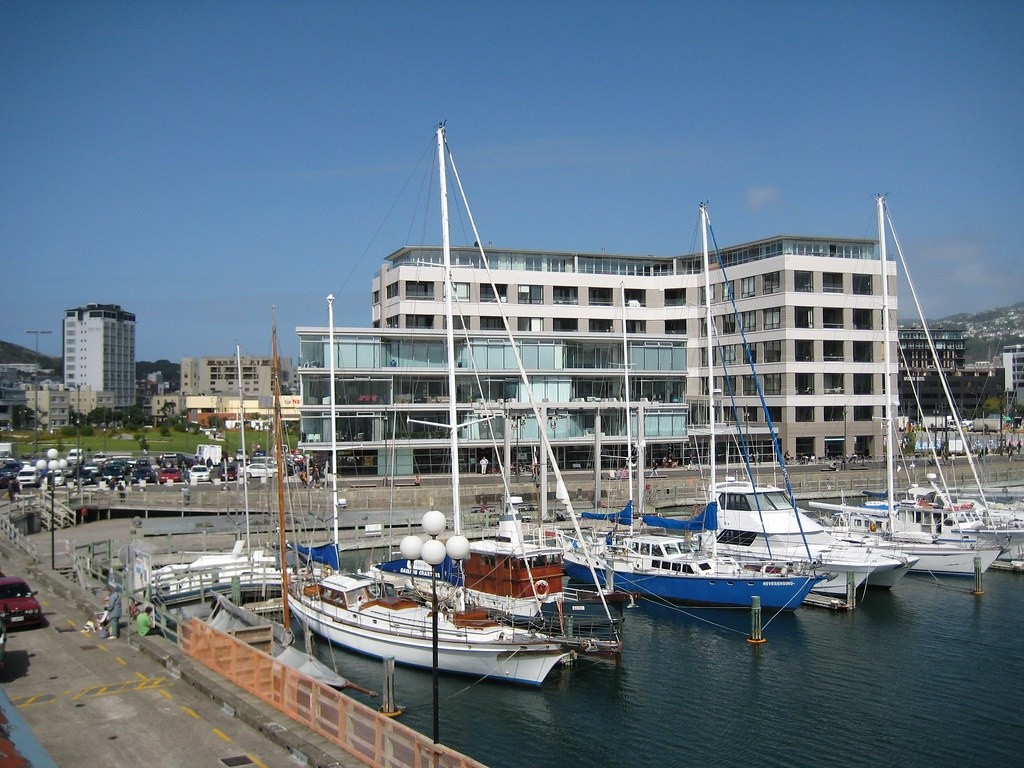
[108,635,116,640]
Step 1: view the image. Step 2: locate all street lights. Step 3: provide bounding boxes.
[68,382,80,494]
[35,449,70,571]
[25,328,53,453]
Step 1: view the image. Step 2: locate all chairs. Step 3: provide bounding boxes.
[306,433,321,443]
[572,398,617,402]
[359,393,379,404]
[640,398,648,402]
[476,398,516,403]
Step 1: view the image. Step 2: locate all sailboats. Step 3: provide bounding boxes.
[166,120,1023,685]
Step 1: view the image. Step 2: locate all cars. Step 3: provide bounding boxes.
[0,576,42,631]
[219,464,238,482]
[239,463,284,478]
[160,467,181,483]
[233,448,249,461]
[0,442,158,487]
[187,464,211,482]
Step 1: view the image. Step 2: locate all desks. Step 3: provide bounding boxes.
[487,400,496,403]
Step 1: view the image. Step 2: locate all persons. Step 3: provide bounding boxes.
[927,452,937,465]
[755,450,761,465]
[829,462,837,472]
[650,459,659,477]
[414,474,421,486]
[784,450,791,464]
[110,479,125,503]
[1007,441,1013,461]
[662,457,667,466]
[480,455,488,474]
[687,455,692,470]
[8,476,20,501]
[137,607,165,638]
[97,584,122,640]
[620,468,627,477]
[311,463,321,489]
[609,468,617,478]
[179,486,191,504]
[951,452,956,467]
[1017,441,1022,454]
[300,470,307,489]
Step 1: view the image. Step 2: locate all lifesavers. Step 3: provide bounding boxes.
[534,579,549,599]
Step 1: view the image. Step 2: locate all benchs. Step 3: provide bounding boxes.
[821,469,832,471]
[850,467,871,470]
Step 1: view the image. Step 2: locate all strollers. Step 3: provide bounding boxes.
[81,610,108,633]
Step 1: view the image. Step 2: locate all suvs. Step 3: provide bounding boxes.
[160,452,180,469]
